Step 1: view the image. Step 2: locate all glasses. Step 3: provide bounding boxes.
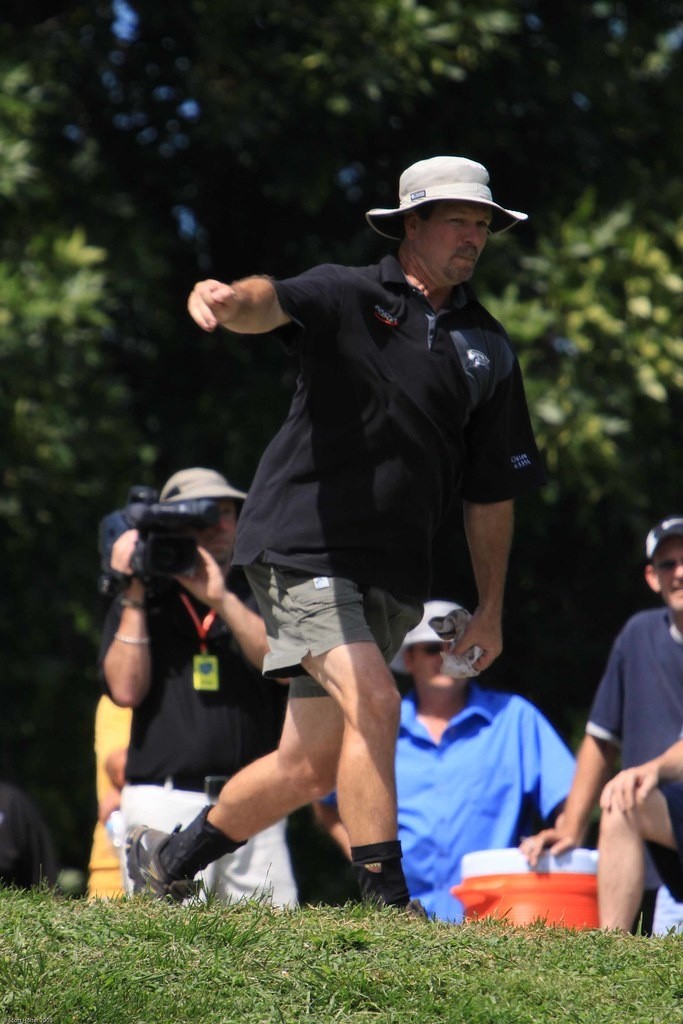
[423,644,442,654]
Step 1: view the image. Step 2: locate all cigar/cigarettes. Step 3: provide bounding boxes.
[521,833,546,856]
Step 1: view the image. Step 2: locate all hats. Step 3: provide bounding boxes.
[646,518,683,557]
[389,600,472,671]
[366,156,528,241]
[159,467,247,502]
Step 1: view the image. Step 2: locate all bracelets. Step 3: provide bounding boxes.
[113,632,153,646]
[120,597,146,608]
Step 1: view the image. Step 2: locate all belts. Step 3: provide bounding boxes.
[125,771,225,796]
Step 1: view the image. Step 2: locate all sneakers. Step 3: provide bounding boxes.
[125,825,203,904]
[405,898,431,923]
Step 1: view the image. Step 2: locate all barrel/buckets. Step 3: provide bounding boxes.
[452,847,599,932]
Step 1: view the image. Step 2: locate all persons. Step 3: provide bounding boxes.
[84,466,310,908]
[520,513,683,933]
[115,154,554,924]
[309,599,583,927]
